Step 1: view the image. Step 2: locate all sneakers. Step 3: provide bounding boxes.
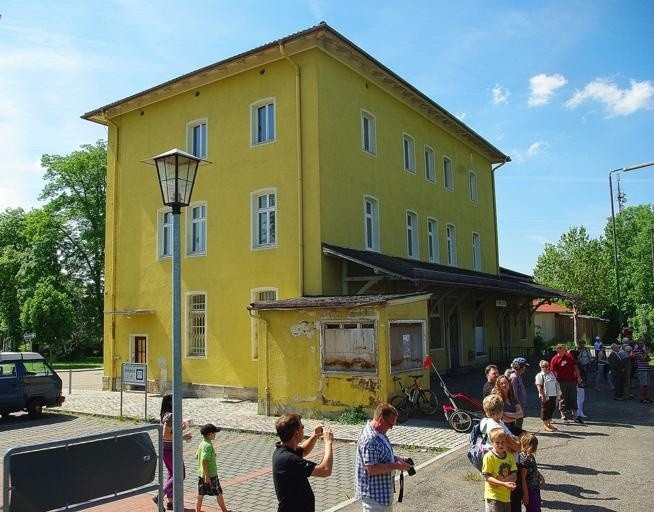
[594,386,653,404]
[539,414,590,431]
[166,502,174,510]
[151,495,165,512]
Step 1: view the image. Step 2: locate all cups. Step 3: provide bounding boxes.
[185,432,191,442]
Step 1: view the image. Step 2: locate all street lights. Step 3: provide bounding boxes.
[607,161,653,345]
[137,147,211,511]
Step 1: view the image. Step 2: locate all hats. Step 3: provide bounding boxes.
[199,423,221,434]
[513,357,530,368]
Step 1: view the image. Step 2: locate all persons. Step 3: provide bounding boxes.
[354,403,412,512]
[469,332,651,512]
[152,393,193,512]
[271,413,334,512]
[194,423,234,512]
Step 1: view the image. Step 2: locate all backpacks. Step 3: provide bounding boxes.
[468,424,486,445]
[468,444,494,473]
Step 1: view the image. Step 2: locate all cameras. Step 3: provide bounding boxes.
[404,457,415,476]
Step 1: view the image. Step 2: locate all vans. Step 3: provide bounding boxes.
[0,352,64,418]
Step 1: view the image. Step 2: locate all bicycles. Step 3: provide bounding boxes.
[389,374,439,424]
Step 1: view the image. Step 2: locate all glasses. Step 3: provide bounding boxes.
[382,417,393,429]
